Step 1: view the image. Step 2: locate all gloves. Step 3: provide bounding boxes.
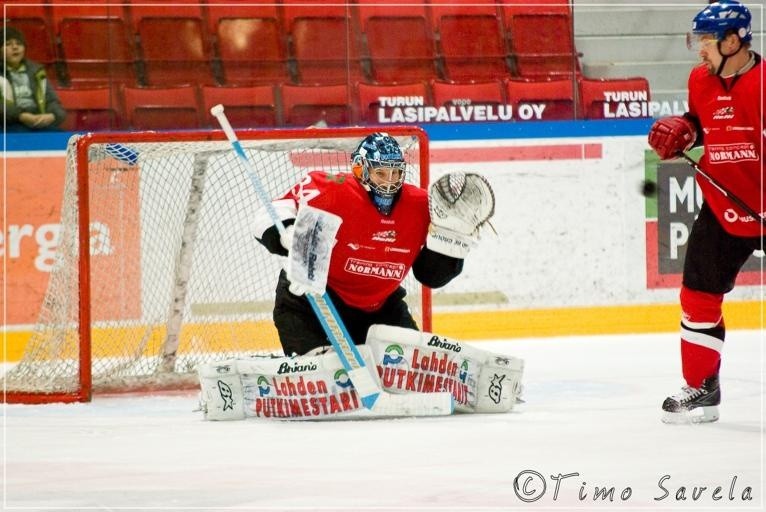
[649,117,695,161]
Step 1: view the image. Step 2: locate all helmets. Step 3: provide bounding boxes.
[686,1,752,52]
[351,132,408,217]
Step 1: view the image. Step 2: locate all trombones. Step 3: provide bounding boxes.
[211,104,455,418]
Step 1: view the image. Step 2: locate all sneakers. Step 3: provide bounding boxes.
[661,375,721,414]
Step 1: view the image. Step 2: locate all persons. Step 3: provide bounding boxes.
[259,133,495,359]
[0,24,67,133]
[648,0,766,424]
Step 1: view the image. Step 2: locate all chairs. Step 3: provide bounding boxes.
[0,0,654,131]
